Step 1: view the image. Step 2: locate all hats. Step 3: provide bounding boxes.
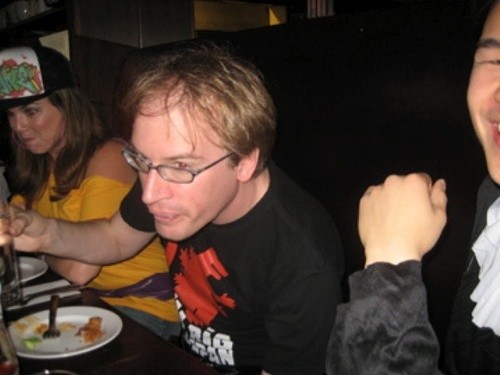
[0,46,79,110]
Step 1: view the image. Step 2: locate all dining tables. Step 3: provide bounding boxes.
[0,252,220,375]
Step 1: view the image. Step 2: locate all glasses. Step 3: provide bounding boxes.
[121,143,237,183]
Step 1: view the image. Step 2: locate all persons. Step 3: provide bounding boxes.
[0,39,345,375]
[1,44,182,342]
[323,1,500,374]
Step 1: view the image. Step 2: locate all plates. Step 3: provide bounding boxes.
[9,256,48,287]
[9,306,123,359]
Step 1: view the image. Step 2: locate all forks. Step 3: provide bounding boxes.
[43,295,61,339]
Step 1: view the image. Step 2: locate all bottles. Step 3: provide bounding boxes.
[0,163,22,309]
[0,304,19,375]
